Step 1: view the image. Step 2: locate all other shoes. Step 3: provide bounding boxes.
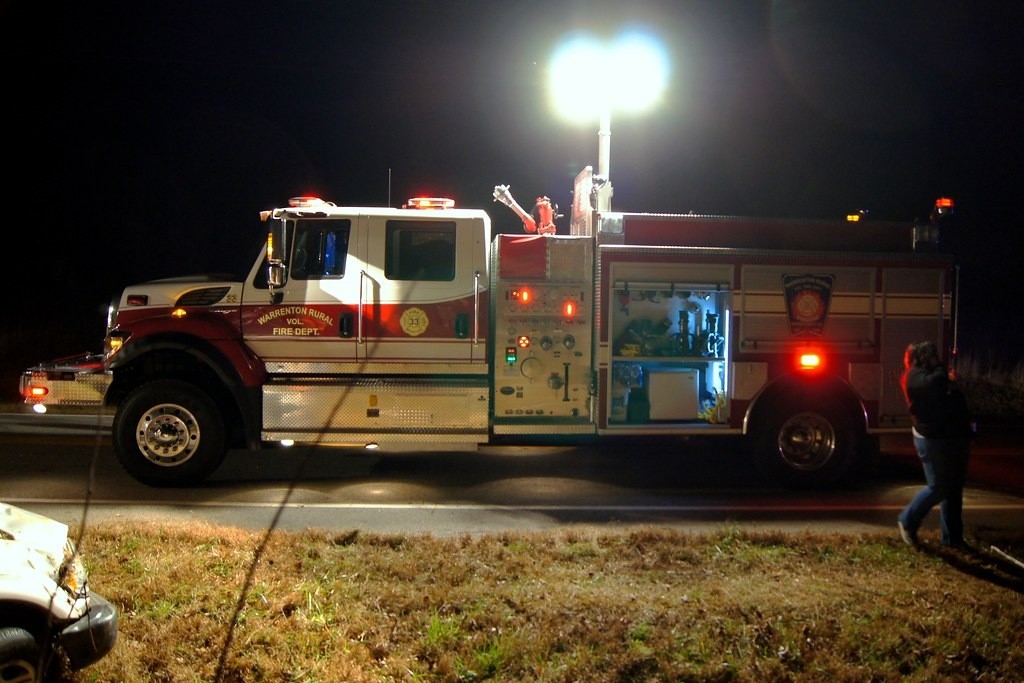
[941,540,970,552]
[898,521,917,546]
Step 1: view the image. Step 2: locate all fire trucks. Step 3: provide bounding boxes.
[97,16,974,501]
[18,351,115,406]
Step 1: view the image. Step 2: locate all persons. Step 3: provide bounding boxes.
[898,340,981,556]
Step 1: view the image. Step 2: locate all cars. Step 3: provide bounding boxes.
[0,500,118,681]
